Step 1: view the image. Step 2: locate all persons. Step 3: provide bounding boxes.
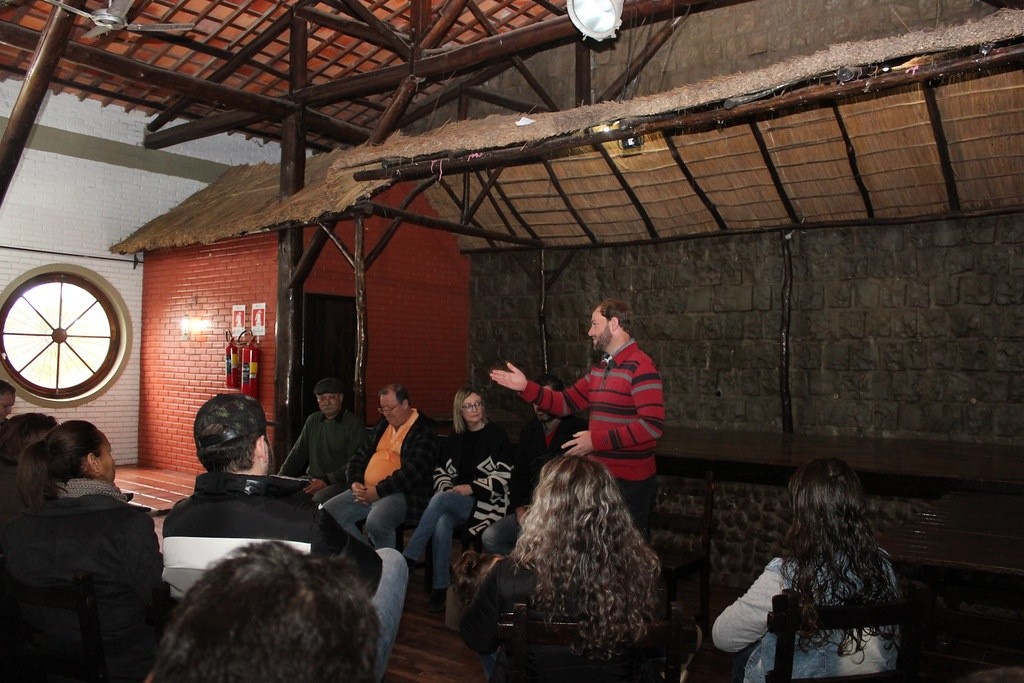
[162,392,410,683]
[445,550,703,683]
[458,455,662,678]
[321,384,439,550]
[0,380,16,425]
[488,298,665,546]
[401,386,516,613]
[277,377,369,494]
[711,456,901,683]
[0,413,59,526]
[143,539,385,683]
[0,420,180,683]
[481,373,589,556]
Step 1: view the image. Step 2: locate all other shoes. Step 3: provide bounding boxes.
[403,555,417,576]
[427,589,445,612]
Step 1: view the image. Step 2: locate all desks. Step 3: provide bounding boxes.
[873,491,1024,669]
[112,464,197,516]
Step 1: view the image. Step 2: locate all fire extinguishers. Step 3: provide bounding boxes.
[237,330,260,401]
[225,329,241,389]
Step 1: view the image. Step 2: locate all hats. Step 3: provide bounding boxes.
[193,393,284,449]
[313,379,345,395]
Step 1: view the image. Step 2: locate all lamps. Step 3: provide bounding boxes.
[566,0,625,44]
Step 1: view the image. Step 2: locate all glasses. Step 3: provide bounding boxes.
[460,400,483,413]
[317,395,340,403]
[376,402,401,415]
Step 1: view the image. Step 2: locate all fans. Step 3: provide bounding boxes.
[42,0,196,41]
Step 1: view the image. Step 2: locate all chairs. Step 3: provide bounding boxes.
[642,470,716,640]
[0,574,108,683]
[496,601,697,683]
[396,435,449,568]
[764,581,929,683]
[423,442,519,594]
